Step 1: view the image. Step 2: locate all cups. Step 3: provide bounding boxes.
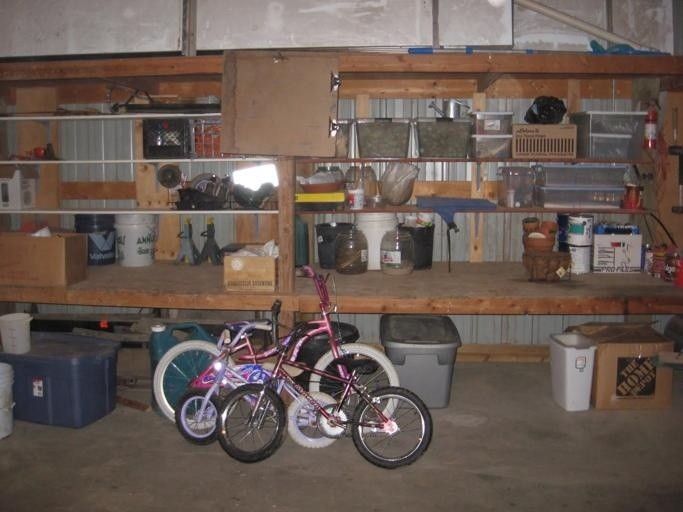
[622,182,643,209]
[347,189,364,210]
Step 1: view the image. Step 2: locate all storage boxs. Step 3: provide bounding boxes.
[468,134,514,161]
[221,243,275,292]
[511,123,577,160]
[0,332,126,429]
[356,118,411,158]
[591,233,644,275]
[470,113,514,135]
[335,120,353,157]
[415,118,473,157]
[0,231,90,288]
[577,317,674,413]
[568,112,646,162]
[529,159,628,209]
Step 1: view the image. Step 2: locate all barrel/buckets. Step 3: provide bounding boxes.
[565,215,594,247]
[397,223,435,270]
[295,213,308,269]
[315,223,353,269]
[354,213,398,270]
[0,362,16,441]
[150,322,222,417]
[74,215,115,265]
[0,313,33,354]
[569,246,589,273]
[113,214,156,267]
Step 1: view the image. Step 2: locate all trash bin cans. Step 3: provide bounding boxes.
[551,333,597,411]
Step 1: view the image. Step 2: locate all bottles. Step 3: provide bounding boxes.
[377,227,417,277]
[332,228,369,275]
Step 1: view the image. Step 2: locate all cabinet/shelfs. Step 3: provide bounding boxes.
[0,52,678,366]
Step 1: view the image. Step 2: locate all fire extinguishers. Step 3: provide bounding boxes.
[644,99,661,149]
